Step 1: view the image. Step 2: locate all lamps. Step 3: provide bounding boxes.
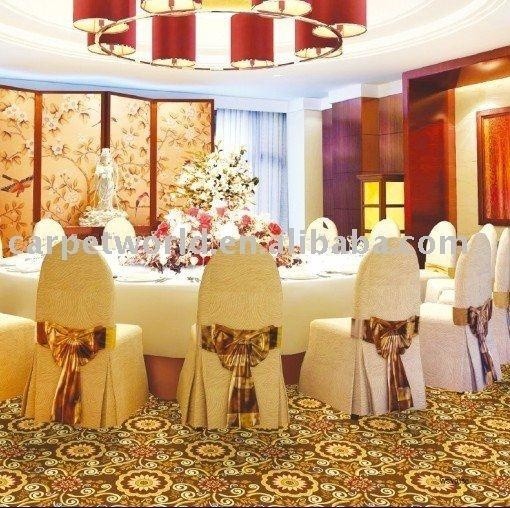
[72,0,367,69]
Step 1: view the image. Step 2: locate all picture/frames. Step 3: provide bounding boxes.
[476,106,510,226]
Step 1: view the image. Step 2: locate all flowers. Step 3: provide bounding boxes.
[124,138,303,274]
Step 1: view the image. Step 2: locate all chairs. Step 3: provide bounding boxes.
[0,218,510,433]
